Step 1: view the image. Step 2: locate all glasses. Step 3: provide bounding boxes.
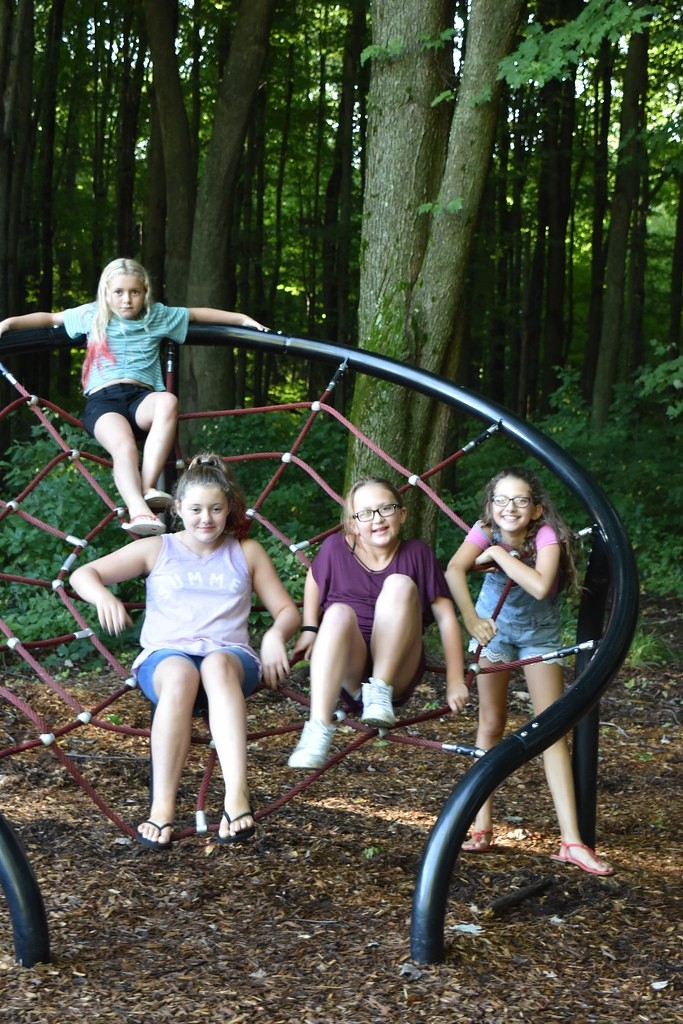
[353,503,402,522]
[490,495,534,508]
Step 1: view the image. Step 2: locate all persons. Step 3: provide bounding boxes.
[443,467,614,876]
[68,452,302,851]
[287,477,470,781]
[0,256,271,536]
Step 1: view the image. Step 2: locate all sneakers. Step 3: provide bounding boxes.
[288,721,336,770]
[359,677,396,729]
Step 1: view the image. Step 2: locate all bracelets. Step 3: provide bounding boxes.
[300,626,318,634]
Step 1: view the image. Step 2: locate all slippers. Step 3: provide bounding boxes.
[216,804,256,844]
[144,489,173,507]
[138,818,174,850]
[121,515,166,535]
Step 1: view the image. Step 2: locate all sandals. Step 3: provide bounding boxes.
[462,829,499,852]
[550,841,615,876]
